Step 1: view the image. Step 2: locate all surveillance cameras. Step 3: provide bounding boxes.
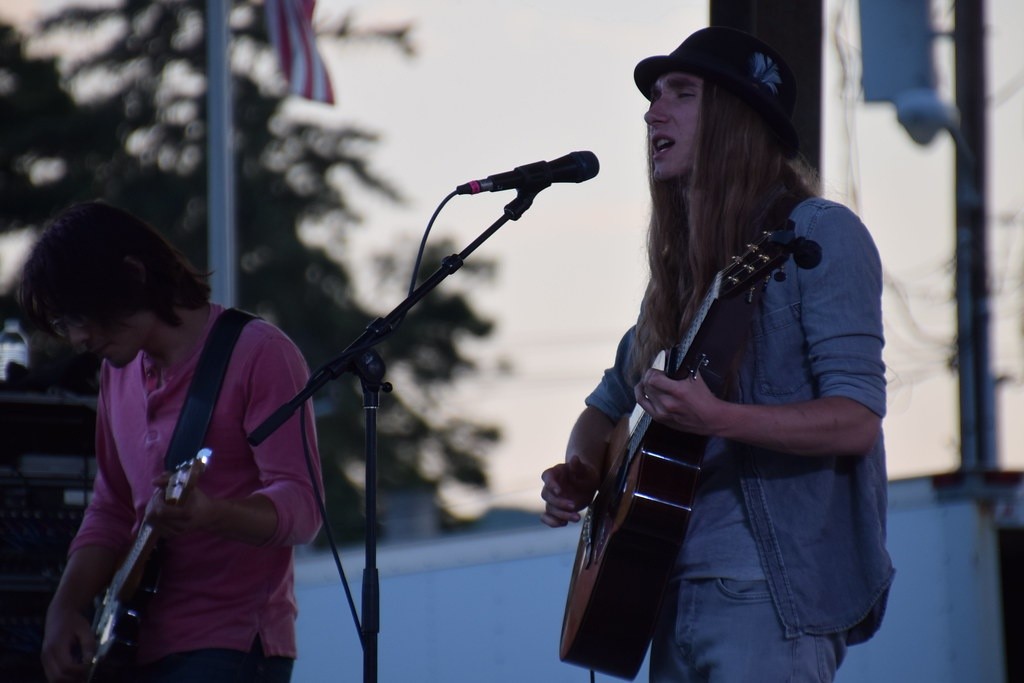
[898,87,944,144]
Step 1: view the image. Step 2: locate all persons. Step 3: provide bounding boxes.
[16,203,328,683]
[541,27,895,682]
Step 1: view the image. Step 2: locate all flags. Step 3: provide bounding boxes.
[266,0,334,106]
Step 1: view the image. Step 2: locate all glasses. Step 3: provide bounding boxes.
[51,313,86,339]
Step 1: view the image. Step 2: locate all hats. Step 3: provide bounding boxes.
[634,27,799,160]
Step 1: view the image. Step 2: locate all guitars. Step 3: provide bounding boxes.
[79,447,220,682]
[557,218,827,679]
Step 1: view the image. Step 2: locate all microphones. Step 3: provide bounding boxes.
[457,151,600,197]
